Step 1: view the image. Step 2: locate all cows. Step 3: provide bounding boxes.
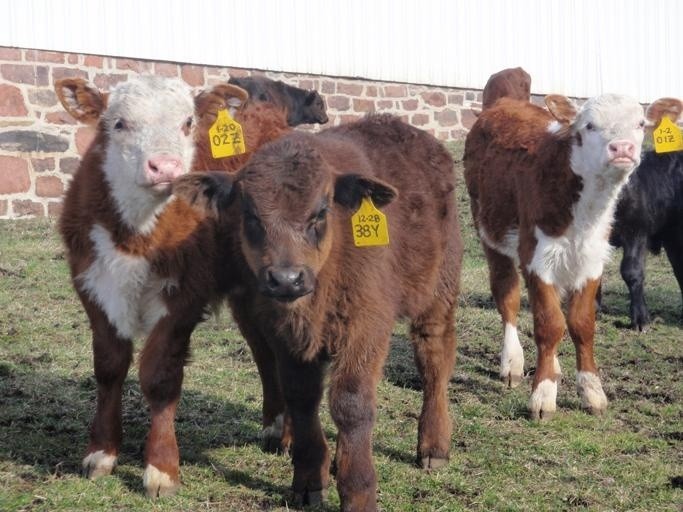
[470,65,532,117]
[224,71,328,130]
[463,95,682,424]
[169,108,466,512]
[52,73,300,501]
